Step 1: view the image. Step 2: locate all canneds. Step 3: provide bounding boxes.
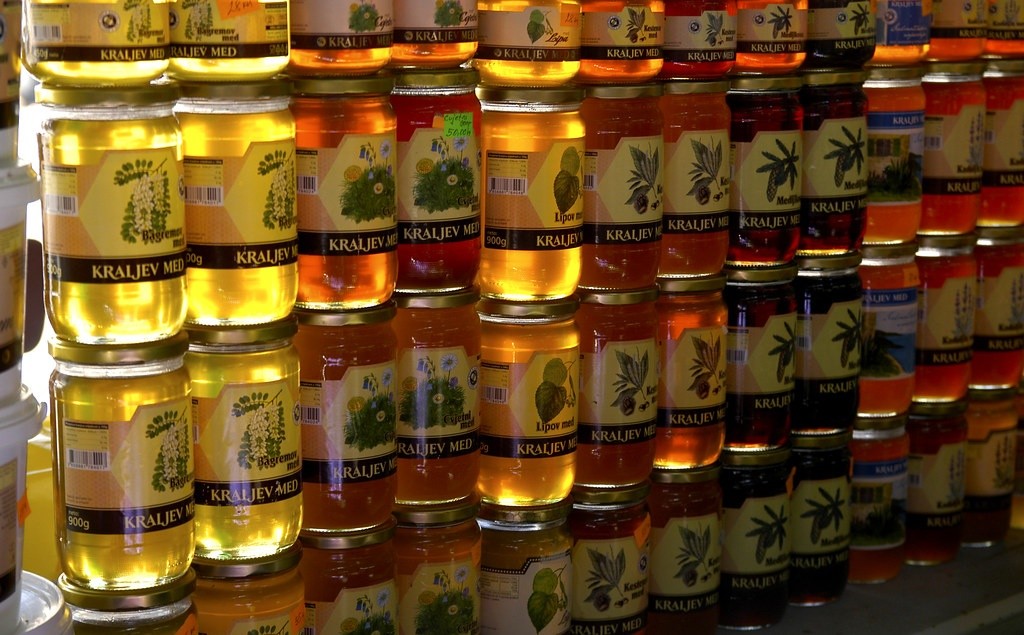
[0,0,1024,635]
